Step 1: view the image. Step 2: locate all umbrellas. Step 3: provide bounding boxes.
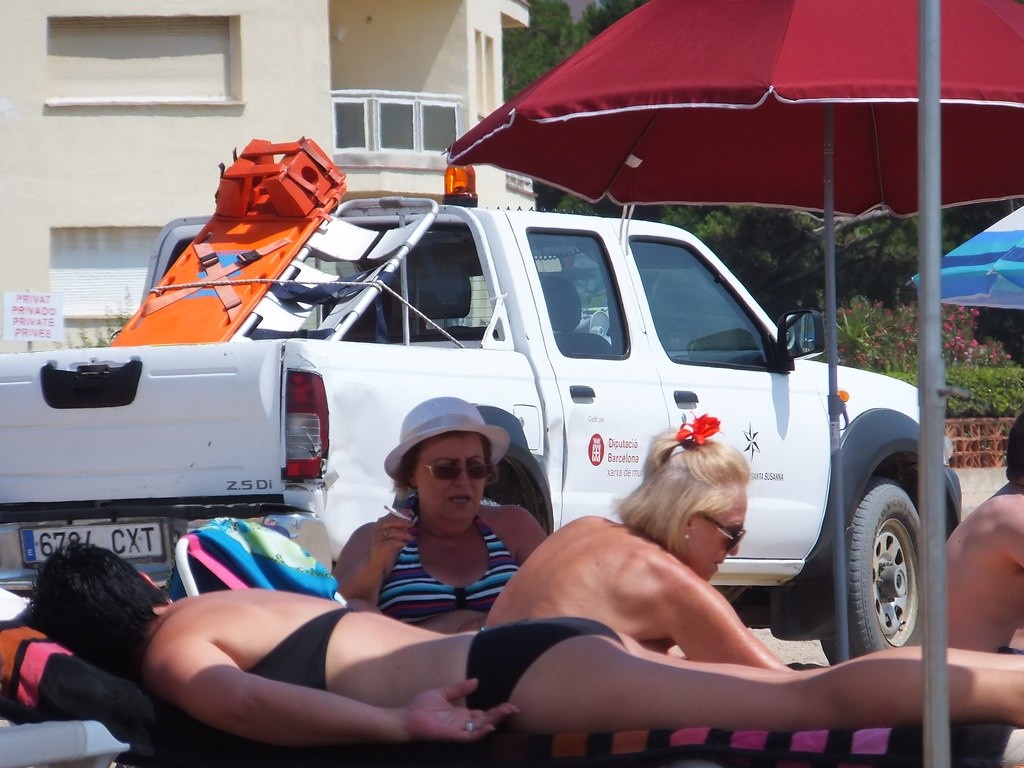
[905,205,1024,309]
[440,0,1024,666]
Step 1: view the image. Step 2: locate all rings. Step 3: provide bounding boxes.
[467,722,471,731]
[384,529,387,537]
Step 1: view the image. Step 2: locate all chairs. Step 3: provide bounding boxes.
[0,519,1024,768]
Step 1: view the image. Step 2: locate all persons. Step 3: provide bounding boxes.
[28,544,1024,744]
[948,411,1024,653]
[487,417,794,672]
[333,396,546,633]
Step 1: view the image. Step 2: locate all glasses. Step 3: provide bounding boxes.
[703,515,748,554]
[421,455,492,480]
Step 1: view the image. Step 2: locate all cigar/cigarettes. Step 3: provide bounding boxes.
[384,506,411,522]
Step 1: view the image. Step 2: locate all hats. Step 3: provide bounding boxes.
[383,396,511,482]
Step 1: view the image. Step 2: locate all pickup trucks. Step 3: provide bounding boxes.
[1,194,964,686]
